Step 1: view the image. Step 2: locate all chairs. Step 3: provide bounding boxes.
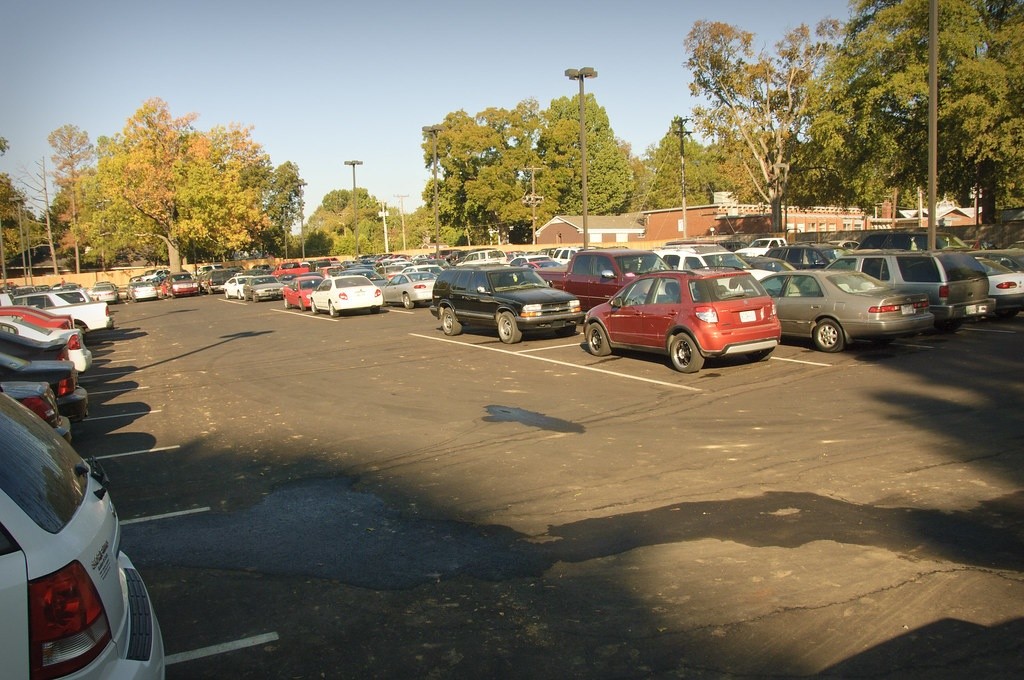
[860,282,875,289]
[787,283,804,297]
[837,284,852,292]
[662,282,679,303]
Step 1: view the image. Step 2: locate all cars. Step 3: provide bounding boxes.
[160,272,199,297]
[761,269,934,351]
[281,275,324,311]
[0,282,167,680]
[581,266,782,372]
[965,240,1024,321]
[308,275,384,317]
[224,247,597,310]
[639,237,857,294]
[382,269,436,309]
[130,269,170,281]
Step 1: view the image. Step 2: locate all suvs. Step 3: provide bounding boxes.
[193,265,223,278]
[202,270,232,295]
[826,251,997,333]
[431,264,587,345]
[852,230,970,252]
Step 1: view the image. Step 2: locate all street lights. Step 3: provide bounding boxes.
[564,67,598,251]
[344,160,363,260]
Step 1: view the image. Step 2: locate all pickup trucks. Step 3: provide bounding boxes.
[536,249,677,311]
[271,262,309,277]
